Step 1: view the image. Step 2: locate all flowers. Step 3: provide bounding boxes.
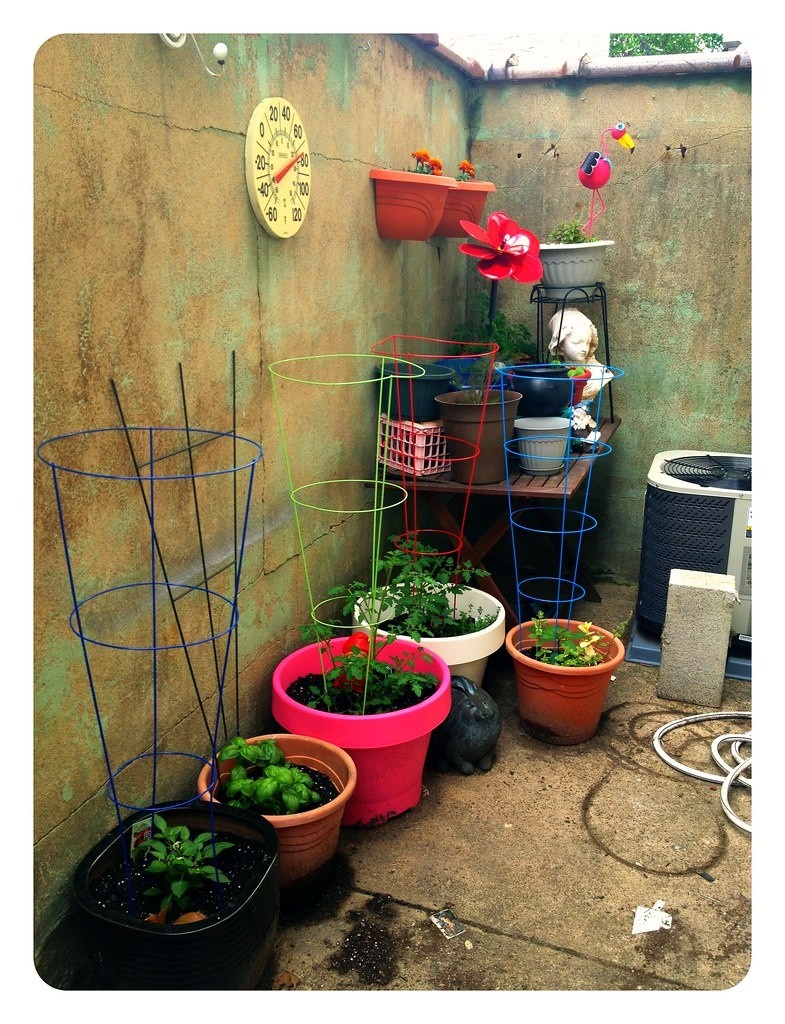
[455,160,475,181]
[410,148,444,176]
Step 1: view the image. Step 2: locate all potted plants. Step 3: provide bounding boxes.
[434,291,532,485]
[351,533,506,688]
[273,580,451,826]
[506,611,633,746]
[73,801,280,990]
[538,220,616,301]
[197,734,356,886]
[551,360,592,406]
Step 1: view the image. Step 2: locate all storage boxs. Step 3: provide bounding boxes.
[377,414,452,476]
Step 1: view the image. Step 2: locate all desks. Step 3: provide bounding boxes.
[363,416,622,622]
[514,417,575,476]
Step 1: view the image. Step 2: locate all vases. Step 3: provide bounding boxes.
[375,362,455,422]
[534,430,539,432]
[430,179,496,238]
[369,168,458,242]
